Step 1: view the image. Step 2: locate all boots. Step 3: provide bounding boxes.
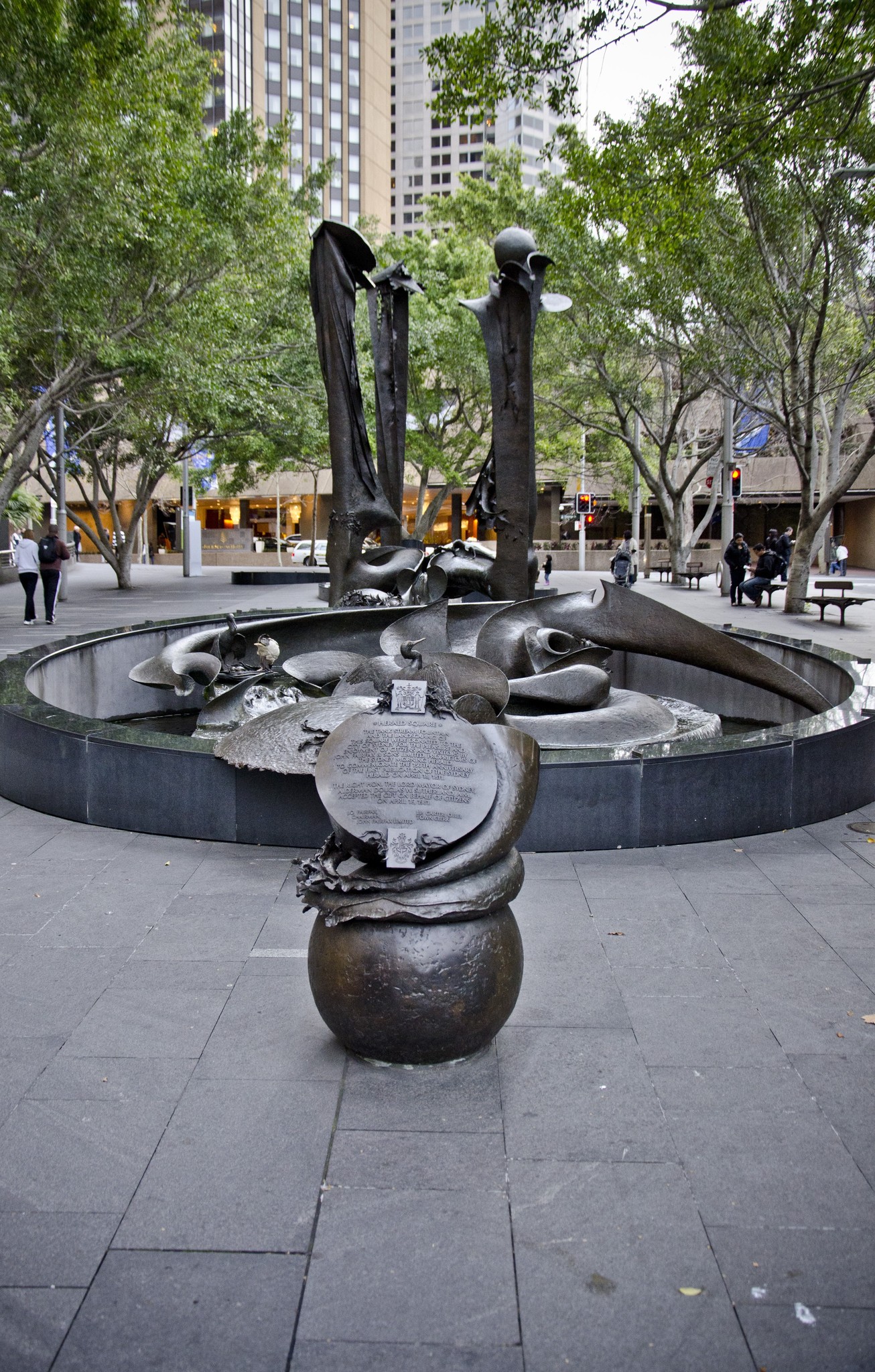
[544,581,549,585]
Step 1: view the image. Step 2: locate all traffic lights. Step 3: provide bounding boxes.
[720,466,741,498]
[577,493,589,513]
[591,492,596,513]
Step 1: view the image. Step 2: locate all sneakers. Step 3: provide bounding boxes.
[52,615,57,621]
[23,620,34,625]
[45,620,55,625]
[31,614,38,621]
[754,595,762,608]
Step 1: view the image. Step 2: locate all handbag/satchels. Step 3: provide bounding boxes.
[77,542,82,552]
[150,551,154,556]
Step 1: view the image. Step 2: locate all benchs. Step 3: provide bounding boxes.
[644,558,672,583]
[754,583,788,608]
[673,561,717,591]
[791,580,875,627]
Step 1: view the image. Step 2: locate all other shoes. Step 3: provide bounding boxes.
[730,602,738,606]
[782,579,788,582]
[737,602,747,606]
[631,583,634,585]
[838,575,845,576]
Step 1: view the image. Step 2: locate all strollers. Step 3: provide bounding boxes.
[613,549,633,589]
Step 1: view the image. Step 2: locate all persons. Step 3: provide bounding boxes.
[148,542,155,564]
[21,528,26,536]
[38,524,70,625]
[776,526,795,582]
[112,525,125,557]
[836,543,848,577]
[72,525,82,562]
[830,542,840,574]
[14,529,40,626]
[142,542,146,564]
[101,528,109,564]
[739,542,775,608]
[770,528,779,550]
[617,529,639,585]
[541,555,553,586]
[11,528,23,549]
[724,533,752,607]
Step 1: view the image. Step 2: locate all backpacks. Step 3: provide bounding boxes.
[38,534,61,563]
[775,535,787,555]
[762,553,787,579]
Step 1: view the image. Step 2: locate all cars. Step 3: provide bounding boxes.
[253,534,301,552]
[292,540,367,566]
[442,537,497,558]
